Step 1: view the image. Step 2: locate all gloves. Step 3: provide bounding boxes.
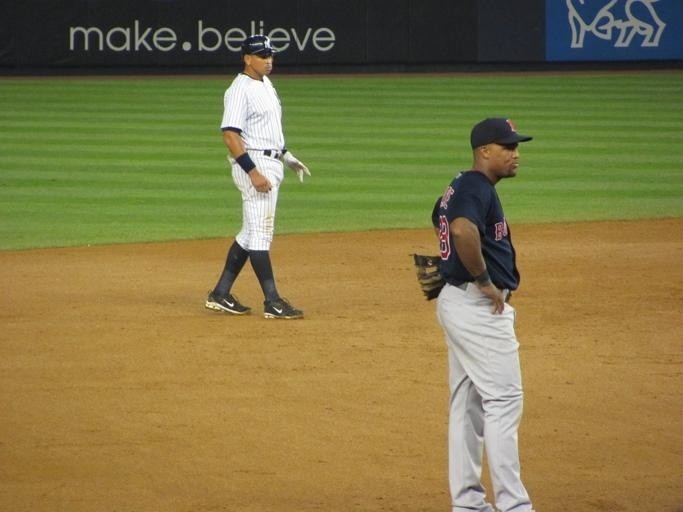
[282,150,311,183]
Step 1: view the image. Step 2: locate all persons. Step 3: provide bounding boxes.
[431,115,537,509]
[204,36,314,321]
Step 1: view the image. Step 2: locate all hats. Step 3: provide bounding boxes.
[471,117,532,149]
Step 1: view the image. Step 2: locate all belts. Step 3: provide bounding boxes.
[265,149,278,158]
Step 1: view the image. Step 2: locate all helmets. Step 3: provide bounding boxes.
[242,34,279,57]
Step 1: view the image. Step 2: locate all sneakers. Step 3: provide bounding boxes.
[205,291,252,315]
[263,297,304,319]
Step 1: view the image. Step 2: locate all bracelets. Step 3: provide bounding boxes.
[473,268,493,288]
[233,152,256,175]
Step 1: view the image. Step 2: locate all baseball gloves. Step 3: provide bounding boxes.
[414,253,446,301]
